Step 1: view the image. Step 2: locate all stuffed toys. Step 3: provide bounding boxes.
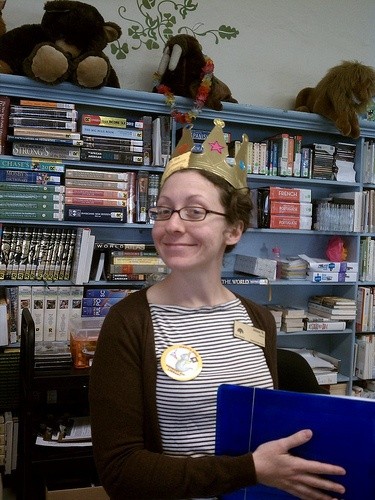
[0,0,12,74]
[295,60,374,139]
[0,0,123,90]
[152,34,238,123]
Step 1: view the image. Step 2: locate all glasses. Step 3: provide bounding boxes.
[145,206,231,222]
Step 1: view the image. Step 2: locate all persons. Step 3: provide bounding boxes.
[88,120,346,500]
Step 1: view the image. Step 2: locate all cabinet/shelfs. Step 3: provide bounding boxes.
[1,77,374,500]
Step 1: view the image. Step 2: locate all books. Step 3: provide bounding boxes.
[0,95,170,476]
[177,127,234,167]
[234,132,375,400]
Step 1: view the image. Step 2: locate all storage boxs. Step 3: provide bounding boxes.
[41,479,110,500]
[69,317,105,369]
